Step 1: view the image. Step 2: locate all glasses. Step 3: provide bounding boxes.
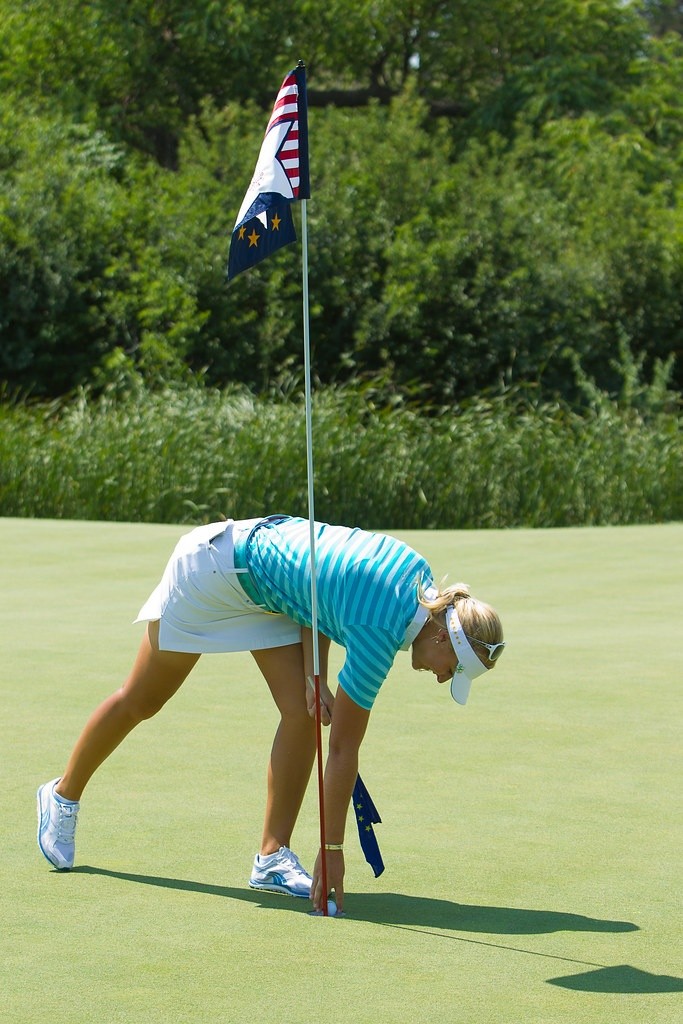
[466,633,505,662]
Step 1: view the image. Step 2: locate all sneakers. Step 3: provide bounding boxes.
[37,777,80,872]
[248,845,332,898]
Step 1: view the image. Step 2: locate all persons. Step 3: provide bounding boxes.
[37,514,505,913]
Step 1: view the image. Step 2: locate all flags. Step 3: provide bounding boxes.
[227,67,310,281]
[353,774,385,879]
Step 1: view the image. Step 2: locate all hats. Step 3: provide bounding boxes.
[446,605,489,705]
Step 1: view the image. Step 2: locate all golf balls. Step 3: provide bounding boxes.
[327,900,337,916]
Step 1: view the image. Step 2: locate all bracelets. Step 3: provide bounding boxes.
[325,844,342,850]
